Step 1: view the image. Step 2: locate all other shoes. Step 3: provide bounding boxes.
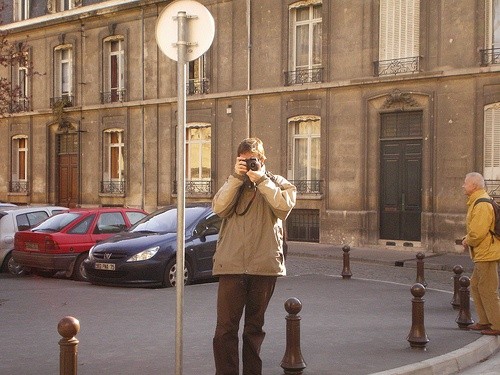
[467,323,491,330]
[482,328,500,335]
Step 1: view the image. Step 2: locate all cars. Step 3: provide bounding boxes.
[0,202,71,277]
[83,202,288,289]
[10,207,154,281]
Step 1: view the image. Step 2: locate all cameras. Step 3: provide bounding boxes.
[244,157,258,171]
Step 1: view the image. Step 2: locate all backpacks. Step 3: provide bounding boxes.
[474,198,500,240]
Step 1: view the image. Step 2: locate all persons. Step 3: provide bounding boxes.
[459,172,500,336]
[211,138,296,375]
